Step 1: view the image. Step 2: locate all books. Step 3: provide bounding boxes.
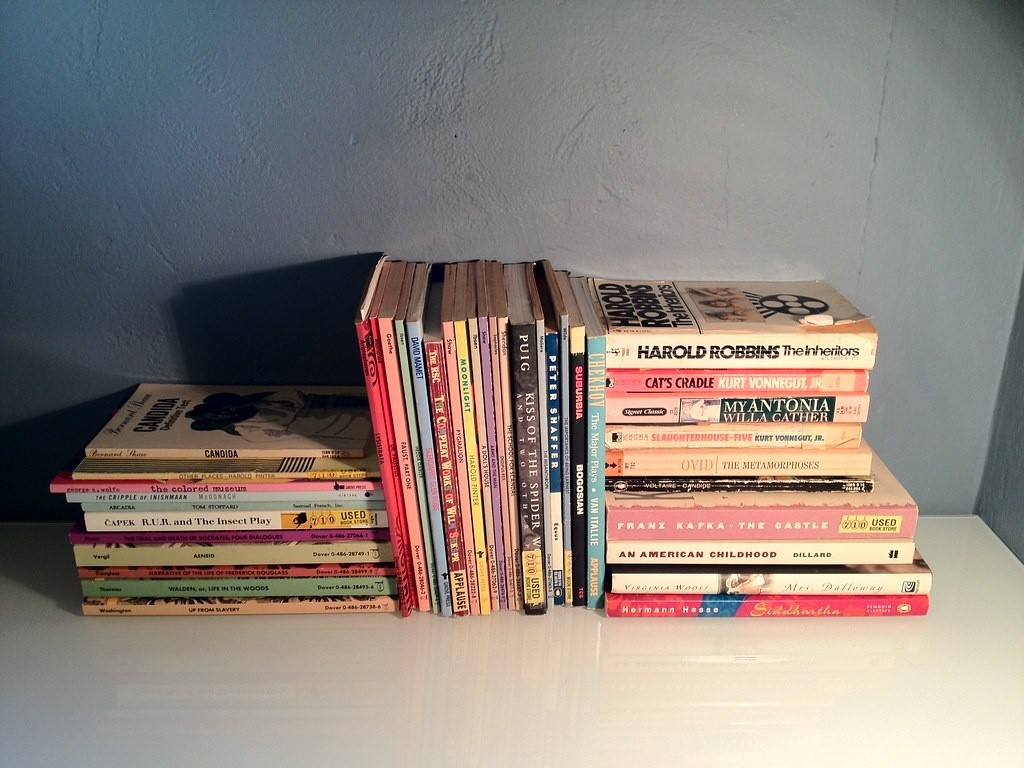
[48,251,935,616]
